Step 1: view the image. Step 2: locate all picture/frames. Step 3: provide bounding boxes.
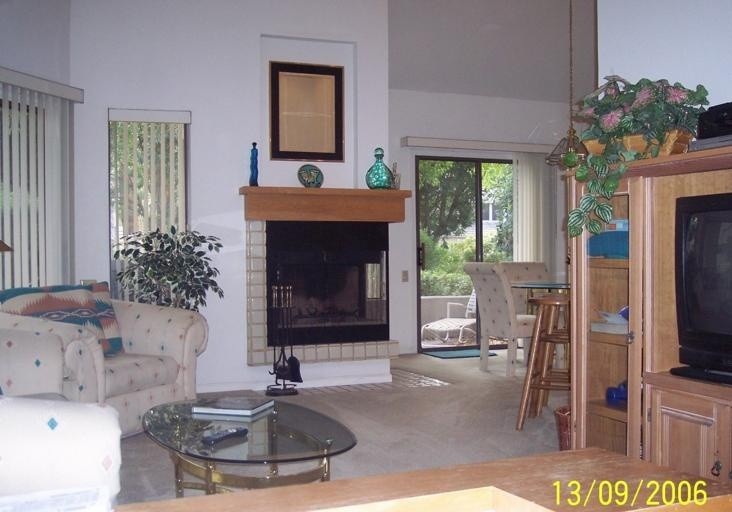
[268,60,344,161]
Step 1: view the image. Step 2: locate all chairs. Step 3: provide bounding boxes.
[0,286,207,446]
[419,260,570,377]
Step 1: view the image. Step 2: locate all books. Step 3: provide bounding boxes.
[592,310,629,335]
[188,394,276,417]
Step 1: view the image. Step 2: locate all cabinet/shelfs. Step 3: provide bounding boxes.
[564,145,732,486]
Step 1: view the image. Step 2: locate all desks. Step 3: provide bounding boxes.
[46,446,732,511]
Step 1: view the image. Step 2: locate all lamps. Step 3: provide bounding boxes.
[544,1,590,170]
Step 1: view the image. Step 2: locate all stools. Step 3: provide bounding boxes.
[516,289,573,430]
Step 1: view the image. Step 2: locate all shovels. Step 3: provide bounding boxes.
[276,286,303,383]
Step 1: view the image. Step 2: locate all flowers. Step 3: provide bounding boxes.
[560,69,707,243]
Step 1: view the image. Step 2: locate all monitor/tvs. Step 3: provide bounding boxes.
[670,193,732,385]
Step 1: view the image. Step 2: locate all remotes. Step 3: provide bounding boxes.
[201,427,248,445]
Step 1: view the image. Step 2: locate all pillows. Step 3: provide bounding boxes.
[1,283,108,353]
[84,277,124,357]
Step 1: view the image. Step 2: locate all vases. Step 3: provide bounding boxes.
[579,129,691,161]
